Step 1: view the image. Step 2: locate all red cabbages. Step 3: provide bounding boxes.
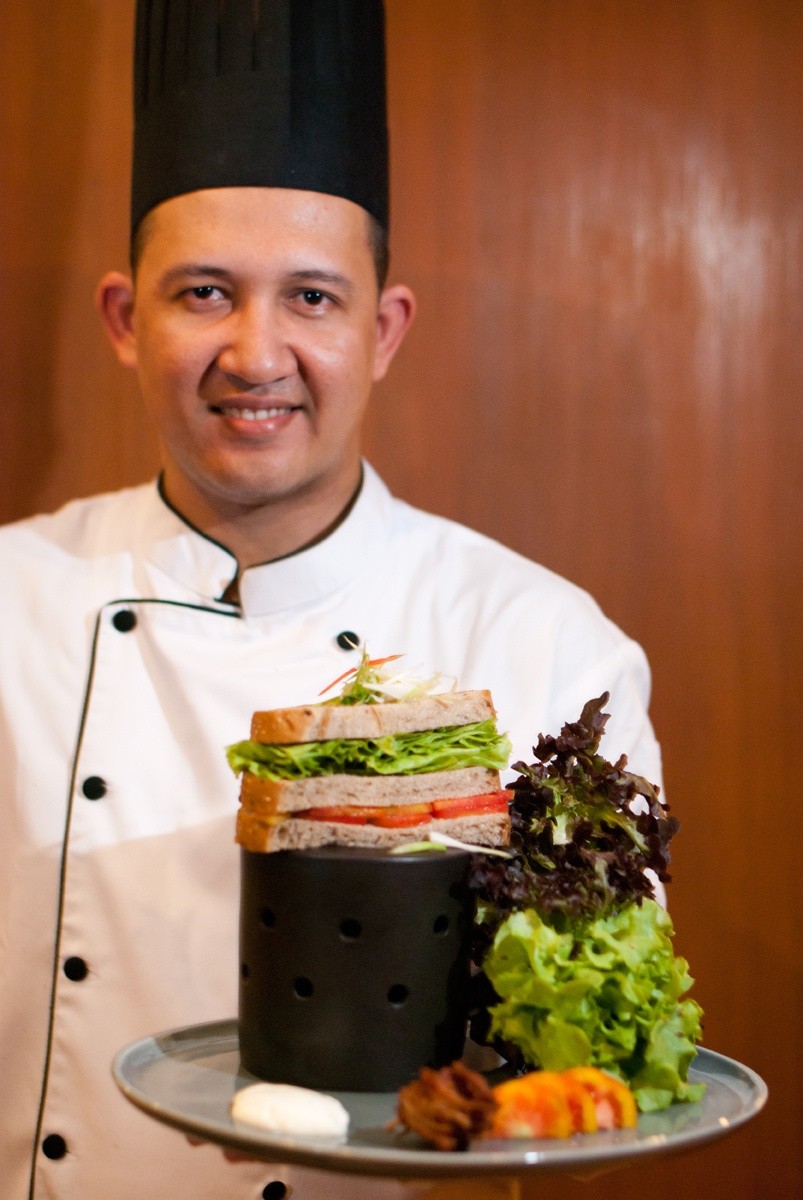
[471,688,709,1111]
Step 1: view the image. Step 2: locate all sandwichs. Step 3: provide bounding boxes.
[231,648,516,856]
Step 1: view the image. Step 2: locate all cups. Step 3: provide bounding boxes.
[234,834,508,1087]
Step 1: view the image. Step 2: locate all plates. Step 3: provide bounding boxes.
[112,1009,768,1175]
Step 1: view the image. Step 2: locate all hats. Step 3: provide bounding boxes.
[131,0,389,230]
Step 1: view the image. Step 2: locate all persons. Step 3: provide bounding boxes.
[0,0,670,1197]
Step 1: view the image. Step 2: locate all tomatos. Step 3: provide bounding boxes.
[479,1068,637,1137]
